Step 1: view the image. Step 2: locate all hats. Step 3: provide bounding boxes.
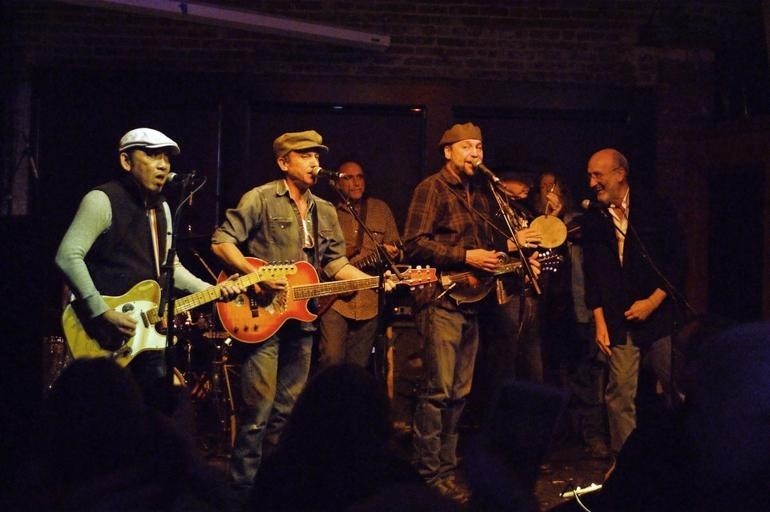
[271,128,331,159]
[436,121,483,146]
[117,127,183,156]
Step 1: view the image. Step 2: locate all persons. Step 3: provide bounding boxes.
[211,122,688,505]
[54,127,247,488]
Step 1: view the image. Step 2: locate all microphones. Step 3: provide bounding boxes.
[167,172,201,187]
[470,158,499,182]
[20,133,40,180]
[312,166,344,178]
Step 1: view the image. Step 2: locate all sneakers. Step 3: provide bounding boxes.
[431,476,474,510]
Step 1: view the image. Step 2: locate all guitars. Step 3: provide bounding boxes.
[438,240,572,305]
[315,231,435,317]
[60,263,299,370]
[215,256,438,345]
